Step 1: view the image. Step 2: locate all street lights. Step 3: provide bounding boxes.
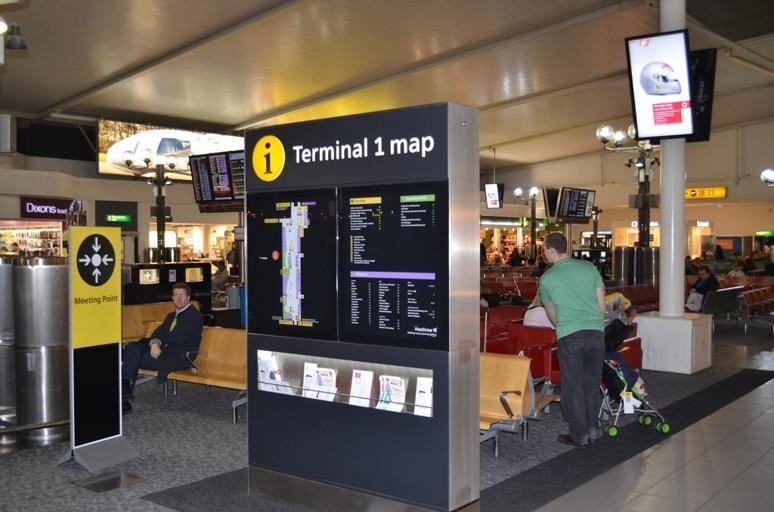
[512,186,540,266]
[596,124,653,245]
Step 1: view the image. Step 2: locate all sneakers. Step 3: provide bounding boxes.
[122,389,135,416]
[558,433,594,447]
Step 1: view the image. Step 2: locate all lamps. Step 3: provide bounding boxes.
[0,23,28,50]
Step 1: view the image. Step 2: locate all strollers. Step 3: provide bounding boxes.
[596,353,671,438]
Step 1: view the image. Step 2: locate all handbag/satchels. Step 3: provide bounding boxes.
[686,293,703,311]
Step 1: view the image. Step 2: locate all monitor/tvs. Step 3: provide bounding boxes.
[555,186,596,219]
[188,149,245,203]
[542,187,559,217]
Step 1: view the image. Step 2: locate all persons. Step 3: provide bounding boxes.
[480,235,529,265]
[540,232,607,448]
[686,236,774,315]
[523,282,638,390]
[211,261,228,290]
[11,243,24,256]
[122,282,204,415]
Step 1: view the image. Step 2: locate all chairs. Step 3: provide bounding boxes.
[211,273,239,304]
[480,264,774,387]
